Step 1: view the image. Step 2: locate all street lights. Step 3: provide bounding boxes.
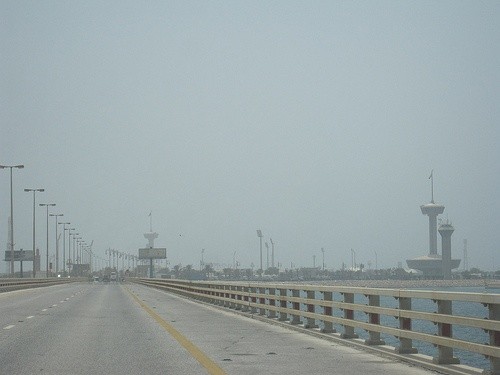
[57,222,70,278]
[81,245,88,275]
[78,243,87,276]
[39,202,55,279]
[77,240,84,276]
[24,187,45,278]
[65,227,76,277]
[49,213,64,278]
[1,163,26,279]
[73,237,82,278]
[69,233,79,277]
